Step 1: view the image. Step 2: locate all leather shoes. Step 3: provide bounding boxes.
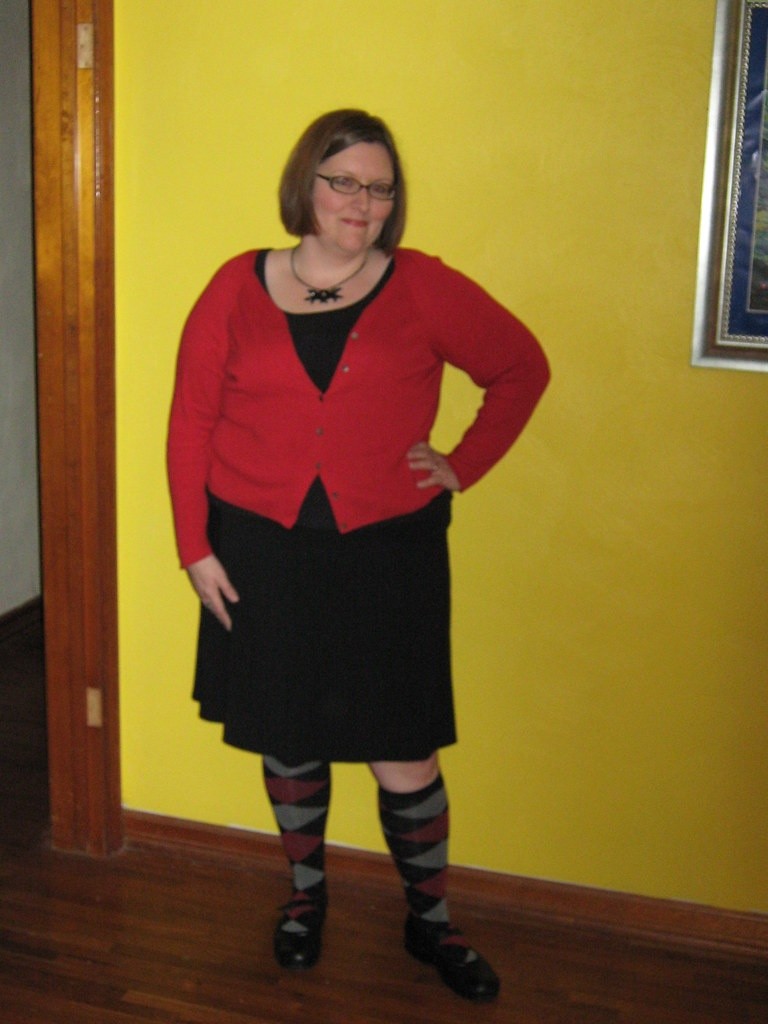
[405,912,500,999]
[274,899,326,970]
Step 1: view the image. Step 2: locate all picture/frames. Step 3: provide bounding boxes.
[689,1,768,374]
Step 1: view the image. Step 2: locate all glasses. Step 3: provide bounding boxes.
[316,173,397,200]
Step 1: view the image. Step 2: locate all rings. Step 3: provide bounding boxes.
[431,463,440,472]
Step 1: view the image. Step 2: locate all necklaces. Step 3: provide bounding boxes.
[290,242,371,302]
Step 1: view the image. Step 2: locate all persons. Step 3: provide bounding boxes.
[165,110,552,1006]
